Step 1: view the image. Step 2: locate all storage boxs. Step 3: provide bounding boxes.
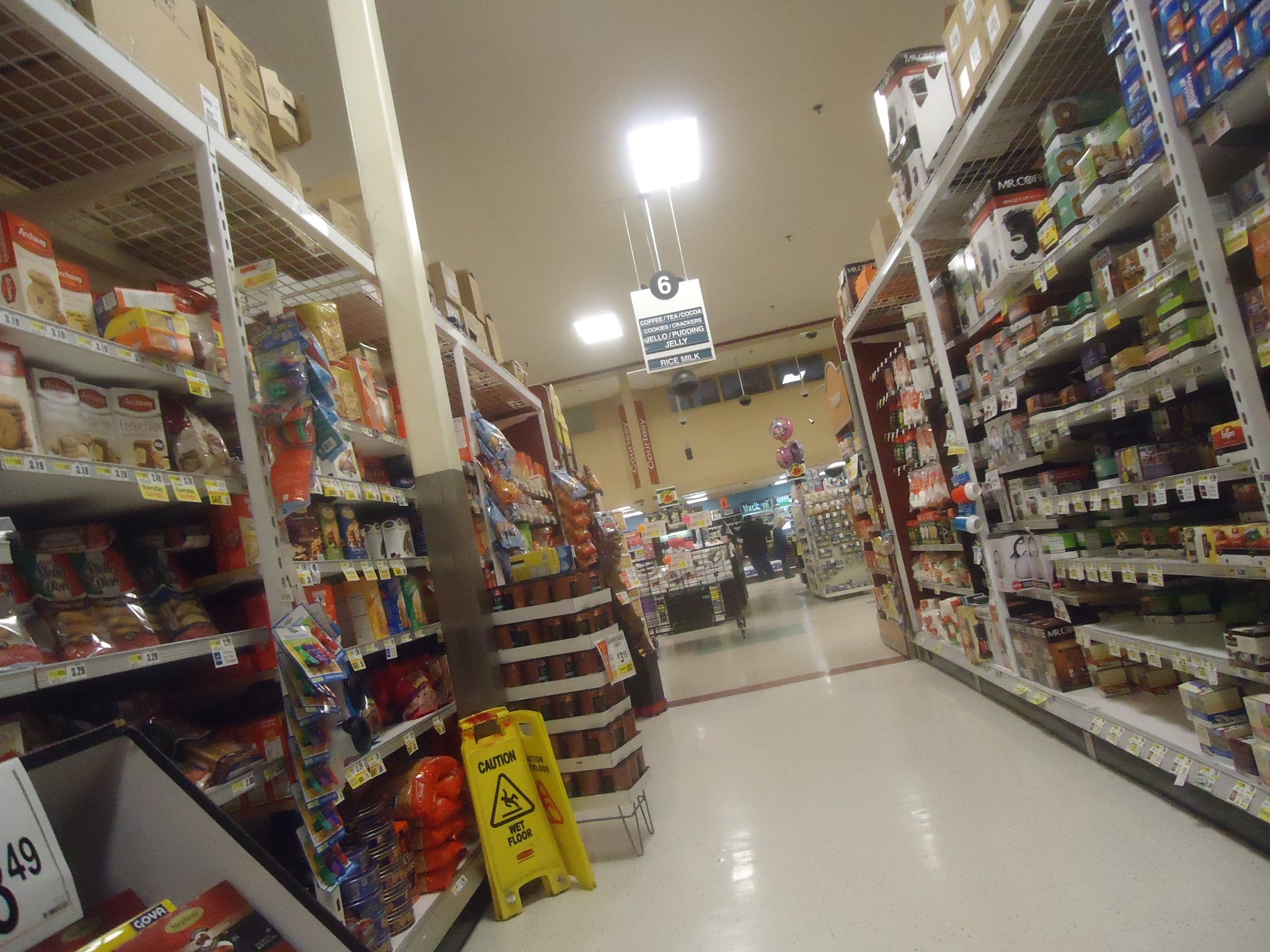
[837,0,1270,785]
[0,0,528,952]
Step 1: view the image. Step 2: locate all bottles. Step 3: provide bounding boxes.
[838,433,855,459]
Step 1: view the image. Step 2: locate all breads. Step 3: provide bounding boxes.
[52,599,208,643]
[1053,102,1085,217]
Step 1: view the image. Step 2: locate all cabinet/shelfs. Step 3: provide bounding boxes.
[492,587,655,858]
[841,0,1270,859]
[822,362,910,657]
[790,484,874,598]
[722,504,799,585]
[0,0,555,952]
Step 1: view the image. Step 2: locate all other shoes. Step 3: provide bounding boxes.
[785,574,795,579]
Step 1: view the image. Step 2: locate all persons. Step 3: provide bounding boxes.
[736,515,776,580]
[774,518,796,579]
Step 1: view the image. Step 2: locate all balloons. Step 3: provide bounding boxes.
[770,416,793,443]
[788,440,804,463]
[776,447,792,471]
